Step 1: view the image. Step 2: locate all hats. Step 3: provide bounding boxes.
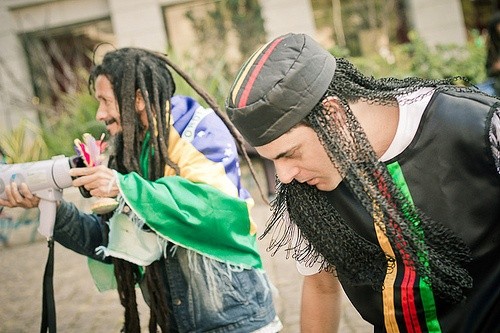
[225,32,336,147]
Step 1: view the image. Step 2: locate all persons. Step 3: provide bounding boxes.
[0,47,284,333]
[485,20,500,81]
[225,34,500,333]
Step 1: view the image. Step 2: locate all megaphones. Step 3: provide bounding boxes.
[0,154,94,240]
